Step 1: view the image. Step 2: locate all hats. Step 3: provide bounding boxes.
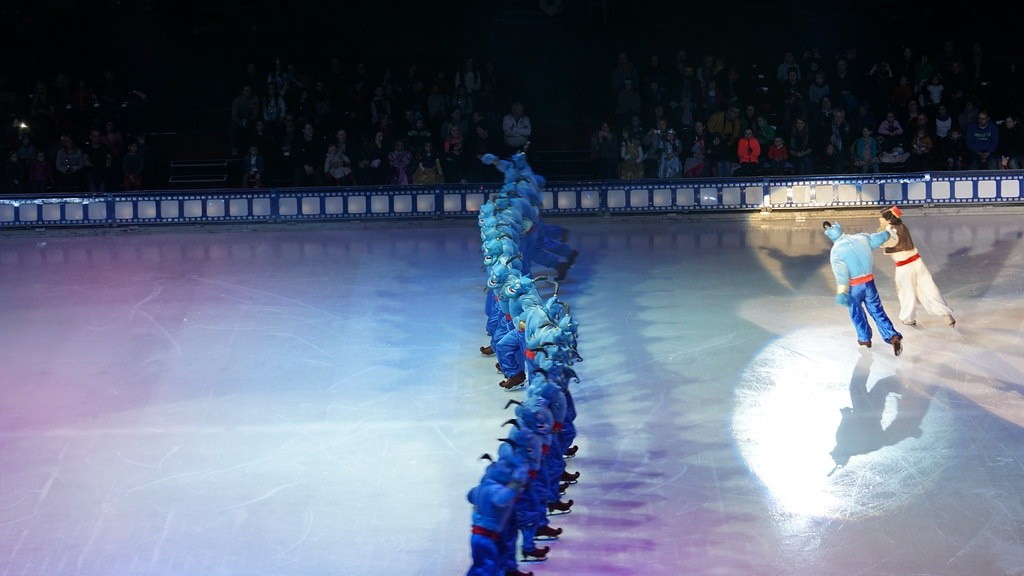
[888,207,902,220]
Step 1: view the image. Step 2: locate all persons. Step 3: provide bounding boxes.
[821,218,904,358]
[469,153,585,575]
[874,206,958,328]
[1,0,1023,175]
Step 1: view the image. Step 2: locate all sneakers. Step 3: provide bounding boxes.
[478,343,526,389]
[522,444,581,562]
[555,227,580,283]
[889,333,902,357]
[943,316,956,328]
[858,339,873,347]
[902,320,916,326]
